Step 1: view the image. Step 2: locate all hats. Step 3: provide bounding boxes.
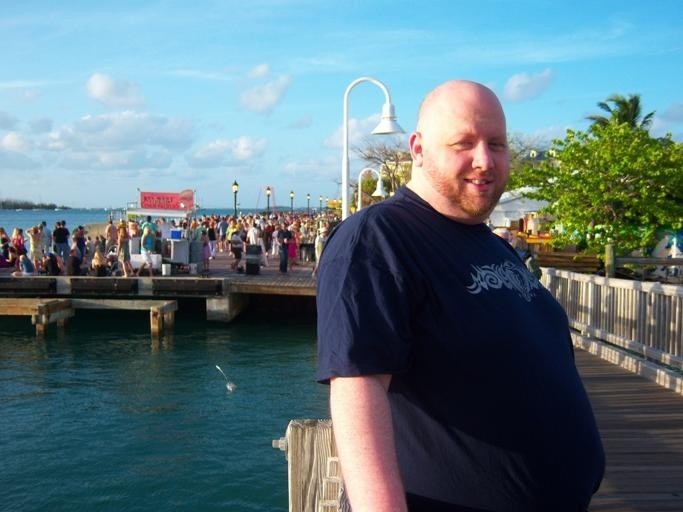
[317,226,328,233]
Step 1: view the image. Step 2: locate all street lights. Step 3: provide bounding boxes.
[307,193,311,216]
[231,180,240,222]
[319,195,322,217]
[289,190,294,216]
[266,187,272,218]
[326,197,330,213]
[342,76,405,220]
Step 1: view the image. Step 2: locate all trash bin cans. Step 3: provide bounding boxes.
[246,245,262,275]
[299,243,314,262]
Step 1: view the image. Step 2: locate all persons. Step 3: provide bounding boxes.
[503,223,543,281]
[493,227,511,243]
[313,78,606,511]
[517,213,582,242]
